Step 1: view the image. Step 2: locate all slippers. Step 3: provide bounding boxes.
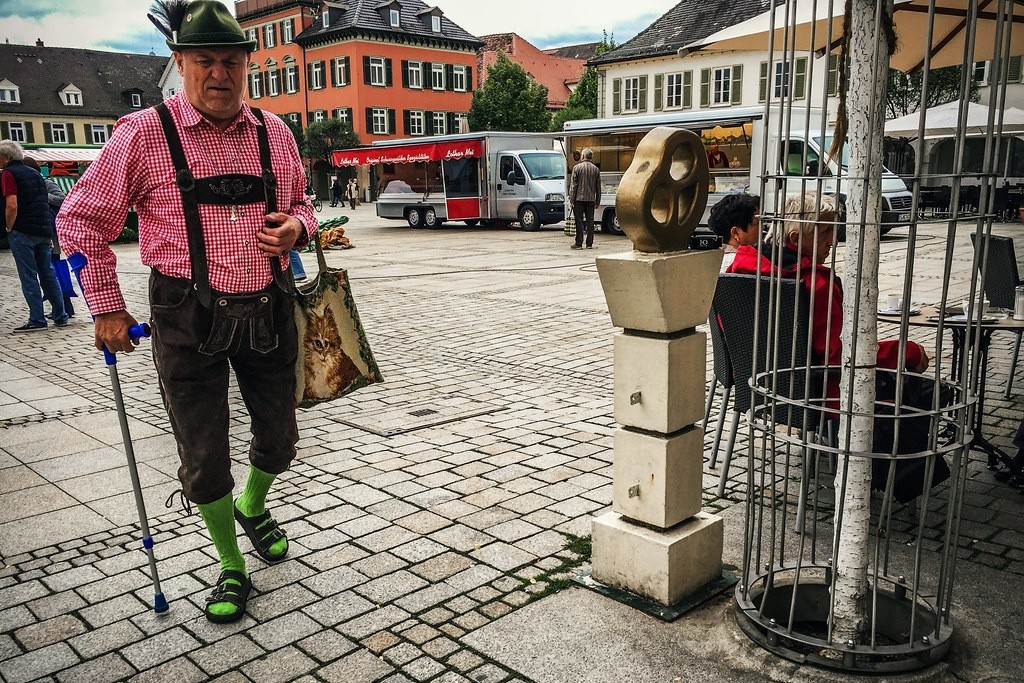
[233,498,289,562]
[205,569,252,623]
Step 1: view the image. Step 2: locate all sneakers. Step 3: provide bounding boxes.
[13,323,48,333]
[53,321,69,327]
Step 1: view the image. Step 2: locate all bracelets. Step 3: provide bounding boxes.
[6,227,12,233]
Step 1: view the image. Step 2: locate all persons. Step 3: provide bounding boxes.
[717,193,950,501]
[22,156,75,319]
[569,148,602,250]
[707,142,729,168]
[329,176,362,211]
[54,1,323,623]
[0,138,68,332]
[706,195,762,277]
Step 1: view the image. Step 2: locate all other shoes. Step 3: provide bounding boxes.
[45,312,75,318]
[570,244,581,250]
[586,244,592,249]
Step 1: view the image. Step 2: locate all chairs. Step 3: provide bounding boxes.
[970,232,1024,399]
[716,273,897,536]
[702,303,734,468]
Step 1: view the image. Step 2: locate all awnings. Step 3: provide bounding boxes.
[331,139,483,167]
[522,112,762,149]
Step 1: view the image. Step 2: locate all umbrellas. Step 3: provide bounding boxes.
[679,0,1024,84]
[883,95,1024,184]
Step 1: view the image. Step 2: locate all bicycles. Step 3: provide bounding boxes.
[309,191,322,212]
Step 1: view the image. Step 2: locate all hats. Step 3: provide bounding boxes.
[166,0,257,52]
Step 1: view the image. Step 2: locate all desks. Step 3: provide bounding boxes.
[877,304,1024,484]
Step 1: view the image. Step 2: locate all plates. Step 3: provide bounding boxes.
[944,315,998,323]
[877,307,922,315]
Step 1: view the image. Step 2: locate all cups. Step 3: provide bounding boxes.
[887,294,903,311]
[962,299,990,320]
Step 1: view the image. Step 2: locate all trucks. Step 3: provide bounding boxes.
[331,132,567,231]
[520,105,913,244]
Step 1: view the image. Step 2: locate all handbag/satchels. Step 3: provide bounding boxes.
[564,207,577,237]
[42,259,78,301]
[292,201,385,409]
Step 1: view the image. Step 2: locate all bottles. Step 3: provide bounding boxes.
[1013,285,1024,320]
